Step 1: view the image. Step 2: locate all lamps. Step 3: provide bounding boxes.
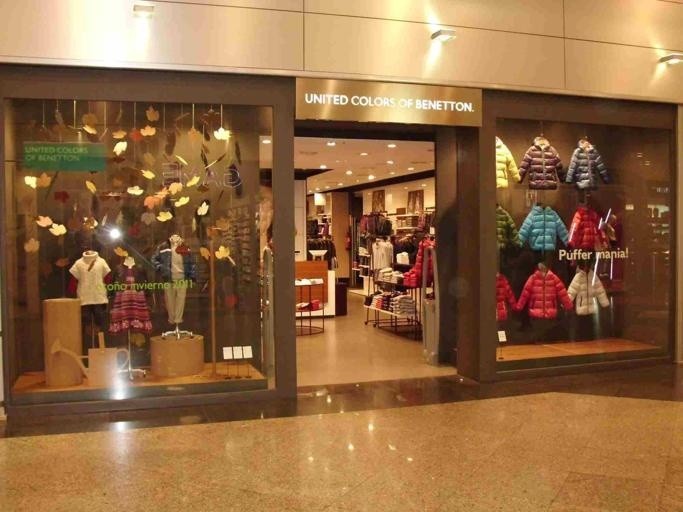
[133,4,154,18]
[660,55,683,64]
[431,30,455,41]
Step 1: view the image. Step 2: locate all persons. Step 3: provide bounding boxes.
[67,251,113,348]
[108,255,153,334]
[150,233,195,324]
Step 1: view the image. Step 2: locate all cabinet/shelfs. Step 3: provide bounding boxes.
[296,214,421,340]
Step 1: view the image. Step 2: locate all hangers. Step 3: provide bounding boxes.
[539,133,593,274]
